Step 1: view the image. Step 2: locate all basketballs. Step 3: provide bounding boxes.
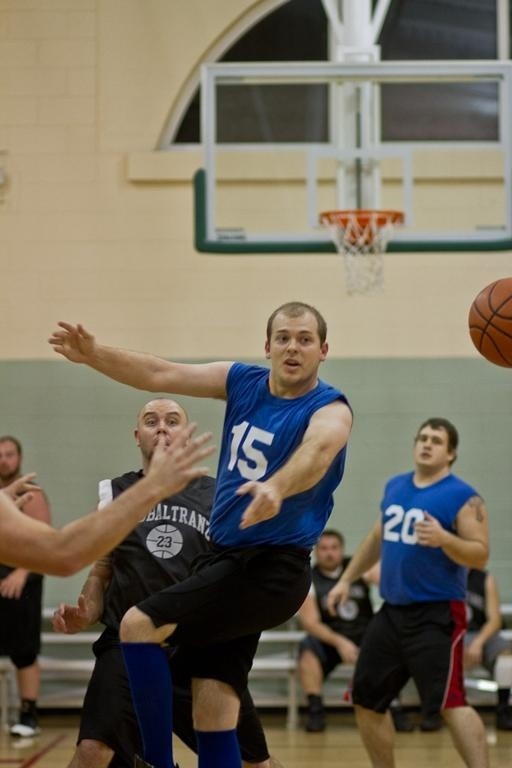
[469,278,511,367]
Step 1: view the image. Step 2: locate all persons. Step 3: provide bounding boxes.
[4,417,218,580]
[299,528,414,734]
[47,298,349,767]
[414,565,512,735]
[3,432,54,744]
[329,416,496,768]
[53,394,282,767]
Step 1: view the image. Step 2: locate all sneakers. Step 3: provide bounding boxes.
[495,712,511,731]
[305,713,326,733]
[392,710,414,732]
[10,724,42,738]
[420,711,440,733]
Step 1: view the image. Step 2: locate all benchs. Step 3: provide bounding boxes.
[0,603,512,731]
